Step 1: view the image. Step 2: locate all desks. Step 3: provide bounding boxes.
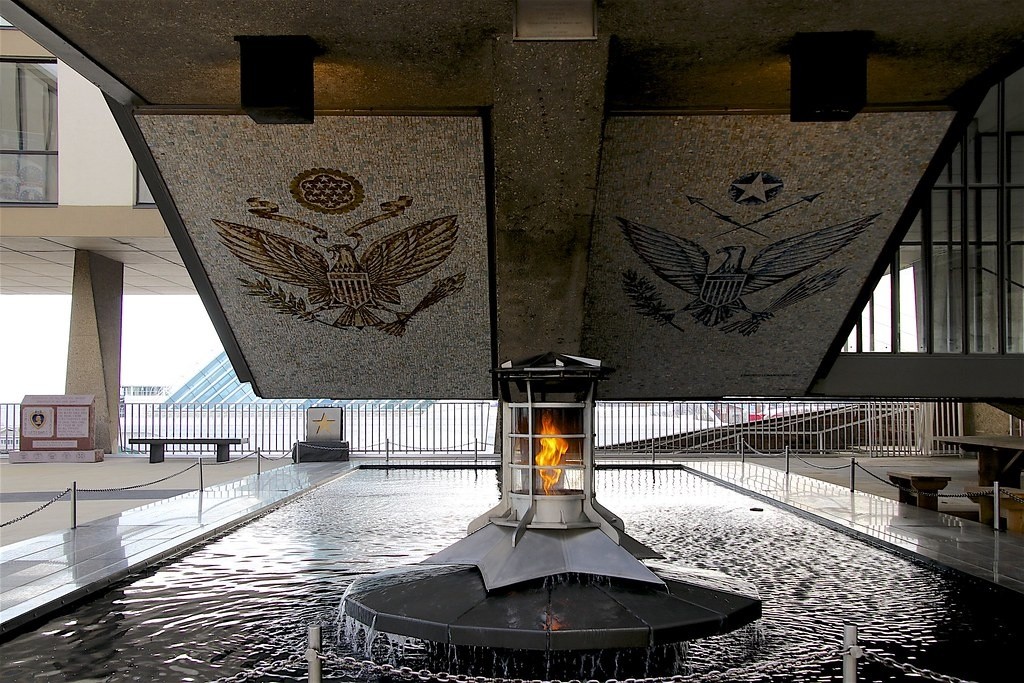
[932,435,1024,489]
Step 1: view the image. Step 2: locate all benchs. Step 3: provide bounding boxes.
[964,485,1024,535]
[129,438,248,461]
[886,469,952,511]
